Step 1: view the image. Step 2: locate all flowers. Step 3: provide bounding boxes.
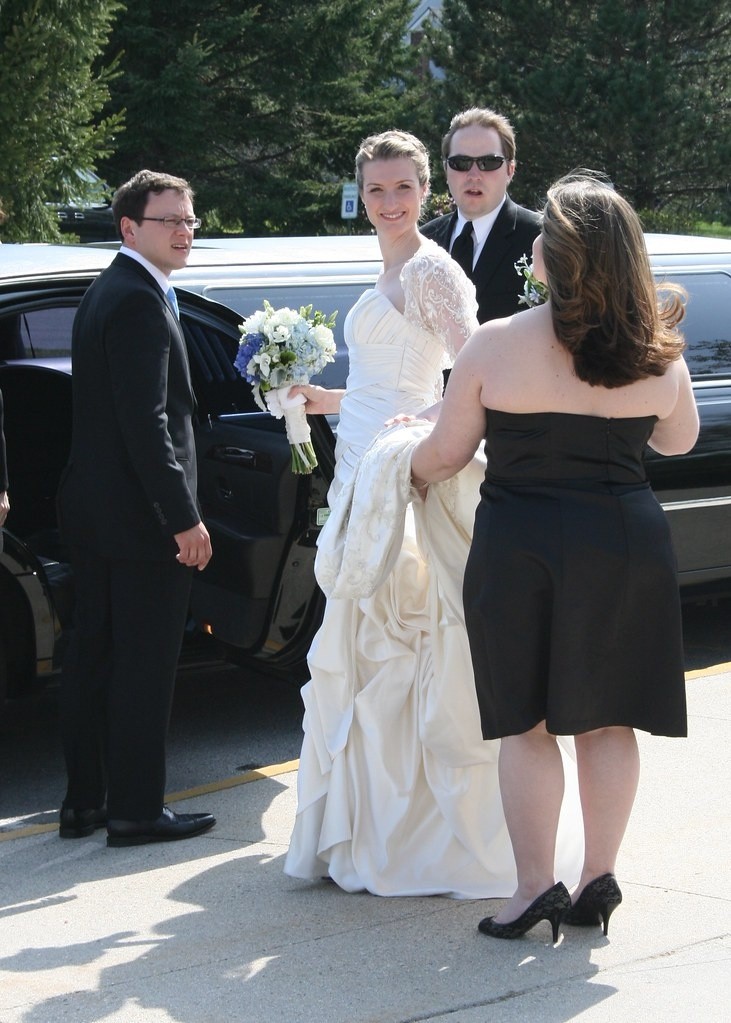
[510,250,551,312]
[232,300,340,475]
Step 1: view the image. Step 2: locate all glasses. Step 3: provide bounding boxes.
[130,216,202,229]
[445,154,506,172]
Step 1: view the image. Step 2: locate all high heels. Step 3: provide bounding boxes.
[568,873,623,935]
[480,879,571,944]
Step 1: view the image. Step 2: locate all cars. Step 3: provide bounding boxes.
[0,204,731,685]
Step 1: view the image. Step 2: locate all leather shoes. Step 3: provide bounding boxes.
[107,806,217,848]
[59,805,111,838]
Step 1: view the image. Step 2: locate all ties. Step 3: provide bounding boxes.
[449,220,476,274]
[167,286,177,307]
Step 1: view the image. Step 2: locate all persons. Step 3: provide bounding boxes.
[414,106,552,400]
[277,129,524,900]
[409,161,706,951]
[44,164,219,848]
